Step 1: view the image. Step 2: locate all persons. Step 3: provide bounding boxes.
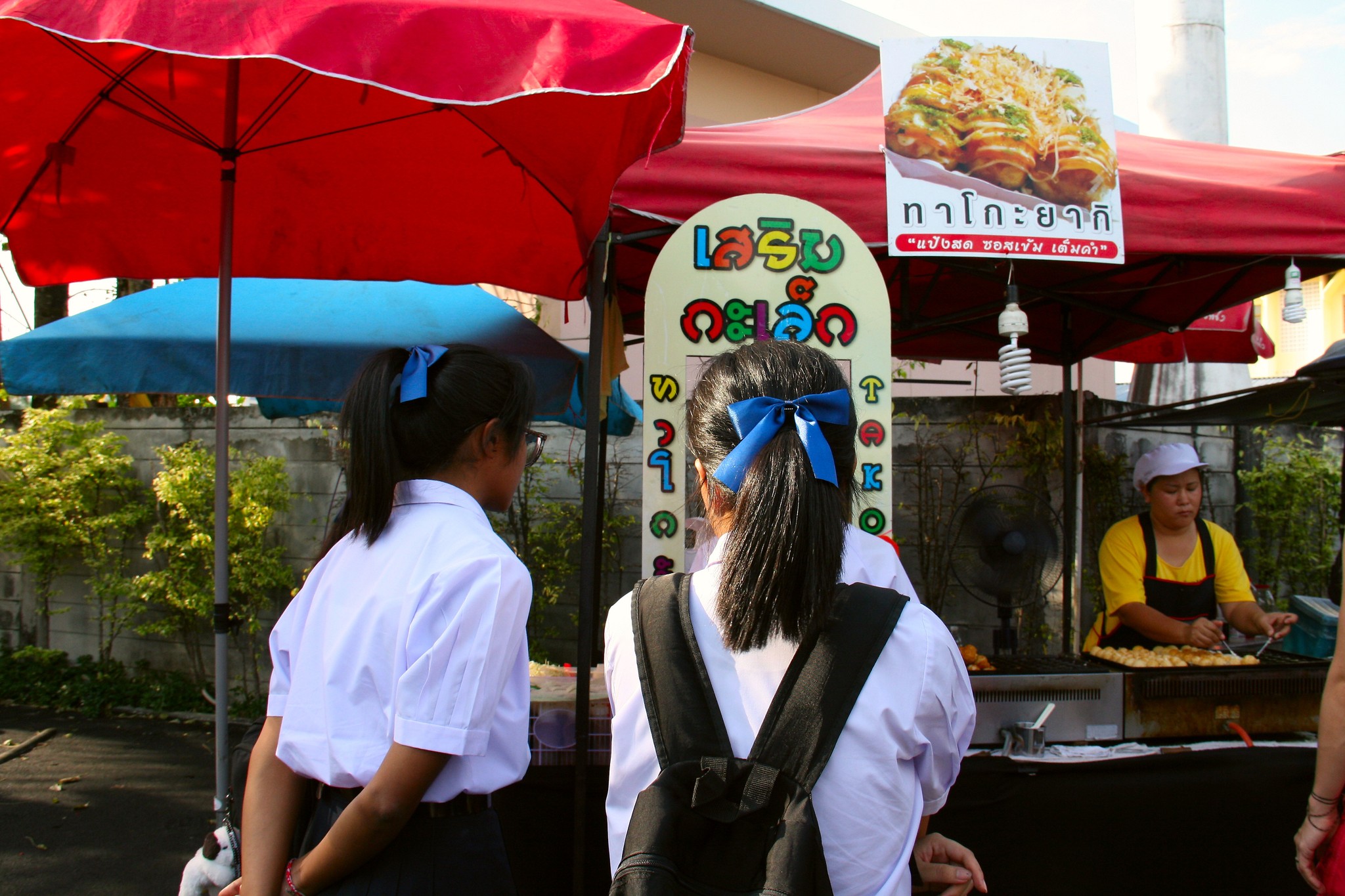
[605,341,988,896]
[1081,441,1297,656]
[220,345,535,896]
[1292,523,1345,896]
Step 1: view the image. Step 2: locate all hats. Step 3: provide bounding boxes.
[1132,443,1209,489]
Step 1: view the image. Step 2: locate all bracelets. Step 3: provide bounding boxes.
[1306,790,1340,832]
[285,859,303,895]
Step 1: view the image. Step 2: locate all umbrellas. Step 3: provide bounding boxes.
[1073,298,1276,651]
[0,2,693,896]
[2,275,643,444]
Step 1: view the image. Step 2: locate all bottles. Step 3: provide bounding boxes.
[1251,584,1275,615]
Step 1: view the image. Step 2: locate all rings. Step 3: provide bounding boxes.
[1294,856,1299,864]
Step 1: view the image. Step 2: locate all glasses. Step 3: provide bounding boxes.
[465,419,547,468]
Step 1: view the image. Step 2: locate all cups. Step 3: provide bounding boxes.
[1013,721,1047,759]
[1229,623,1247,644]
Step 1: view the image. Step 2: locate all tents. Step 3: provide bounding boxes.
[548,59,1345,795]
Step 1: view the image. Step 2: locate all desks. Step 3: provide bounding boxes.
[505,736,1317,896]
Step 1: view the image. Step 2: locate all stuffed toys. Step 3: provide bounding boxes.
[178,825,242,896]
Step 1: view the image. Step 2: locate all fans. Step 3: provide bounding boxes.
[944,485,1072,658]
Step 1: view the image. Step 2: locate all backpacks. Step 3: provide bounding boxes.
[608,571,909,896]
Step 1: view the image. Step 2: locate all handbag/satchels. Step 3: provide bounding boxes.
[223,715,325,863]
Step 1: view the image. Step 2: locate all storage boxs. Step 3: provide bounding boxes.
[1285,594,1340,658]
